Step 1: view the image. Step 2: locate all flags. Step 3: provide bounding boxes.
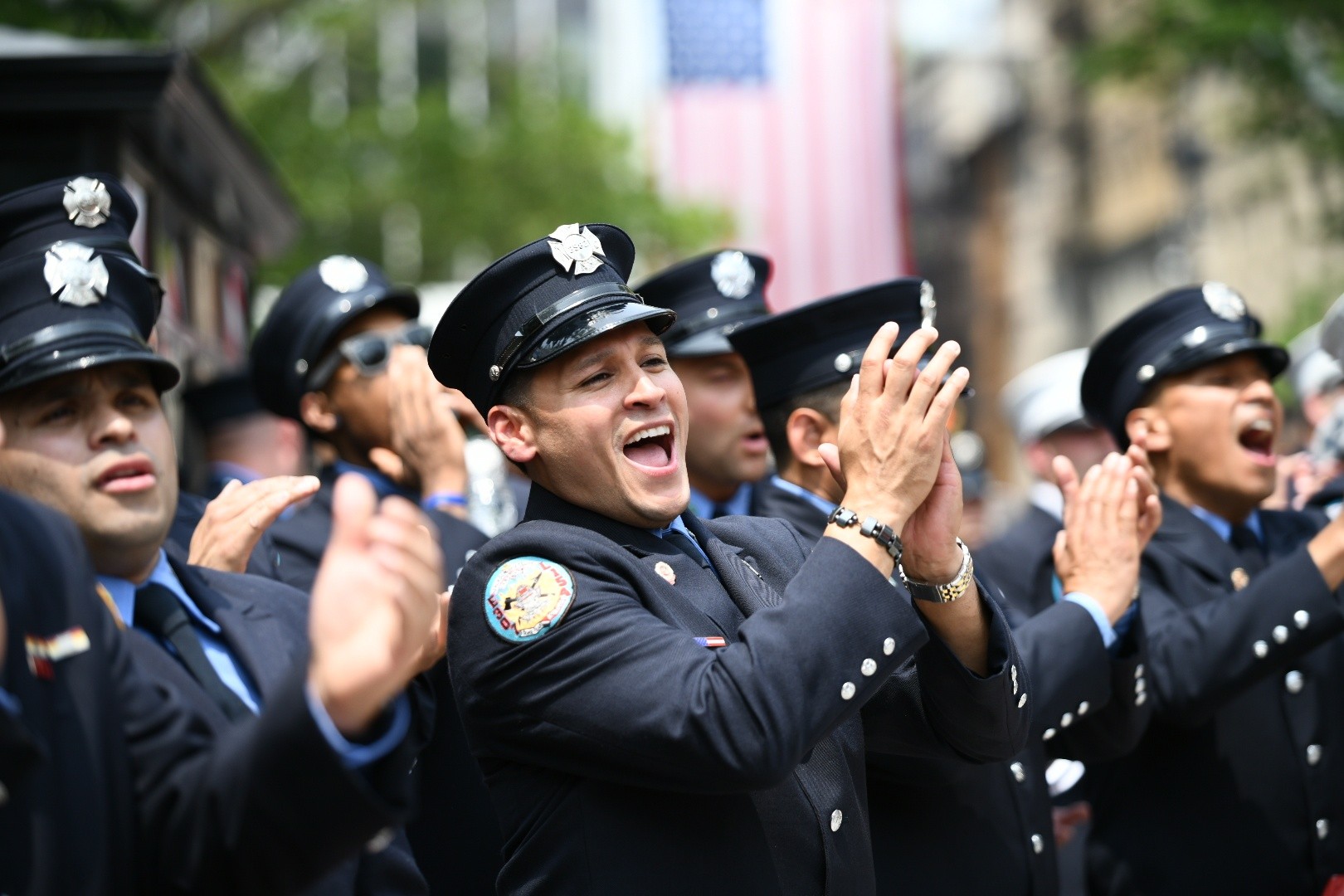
[663,0,783,198]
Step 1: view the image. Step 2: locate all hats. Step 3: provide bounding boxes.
[0,242,180,396]
[999,346,1094,448]
[1,175,143,265]
[246,255,420,418]
[726,278,975,412]
[1286,292,1344,398]
[427,222,675,419]
[635,249,771,355]
[1080,280,1289,436]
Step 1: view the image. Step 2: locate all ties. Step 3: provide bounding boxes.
[1227,522,1269,577]
[133,583,257,728]
[663,534,729,596]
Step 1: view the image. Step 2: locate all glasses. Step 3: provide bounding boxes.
[310,325,432,387]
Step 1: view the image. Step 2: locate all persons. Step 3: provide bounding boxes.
[0,174,1344,896]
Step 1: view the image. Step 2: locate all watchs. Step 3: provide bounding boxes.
[898,537,974,603]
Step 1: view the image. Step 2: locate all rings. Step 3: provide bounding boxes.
[247,519,265,532]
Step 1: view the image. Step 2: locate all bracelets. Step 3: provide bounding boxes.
[827,506,902,568]
